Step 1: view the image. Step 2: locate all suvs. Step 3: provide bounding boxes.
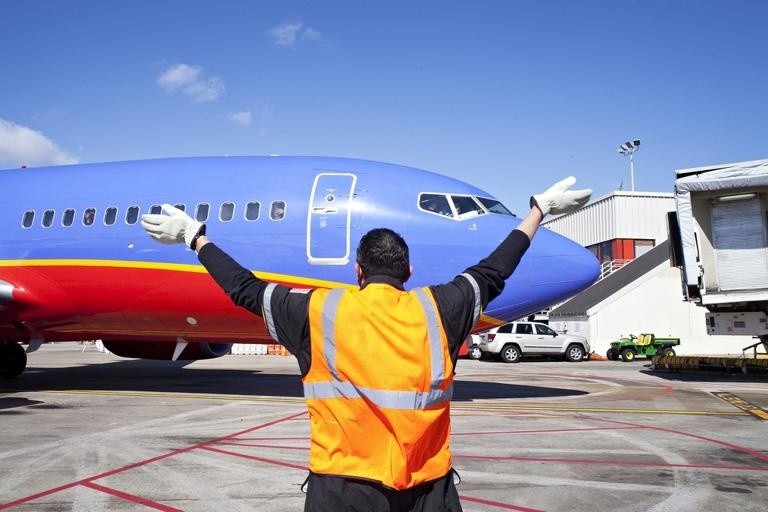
[479,321,590,362]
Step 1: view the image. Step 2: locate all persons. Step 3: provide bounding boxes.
[142,176,593,512]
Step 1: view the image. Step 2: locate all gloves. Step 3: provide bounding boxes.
[531,175,593,220]
[140,204,206,250]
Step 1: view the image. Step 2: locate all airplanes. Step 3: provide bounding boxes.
[1,154,601,384]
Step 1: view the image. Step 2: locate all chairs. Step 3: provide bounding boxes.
[637,334,652,348]
[633,334,645,345]
